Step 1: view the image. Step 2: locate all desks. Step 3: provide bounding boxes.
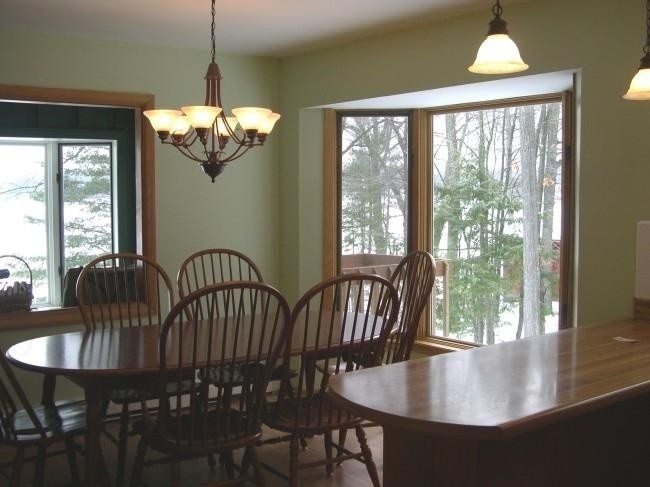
[6,310,400,486]
[327,310,650,485]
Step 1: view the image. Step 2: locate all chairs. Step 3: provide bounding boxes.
[303,250,436,450]
[131,281,292,486]
[75,253,205,462]
[2,350,102,485]
[177,246,299,452]
[238,273,401,485]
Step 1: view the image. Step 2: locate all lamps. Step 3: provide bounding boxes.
[465,1,530,75]
[142,0,283,184]
[620,0,650,101]
